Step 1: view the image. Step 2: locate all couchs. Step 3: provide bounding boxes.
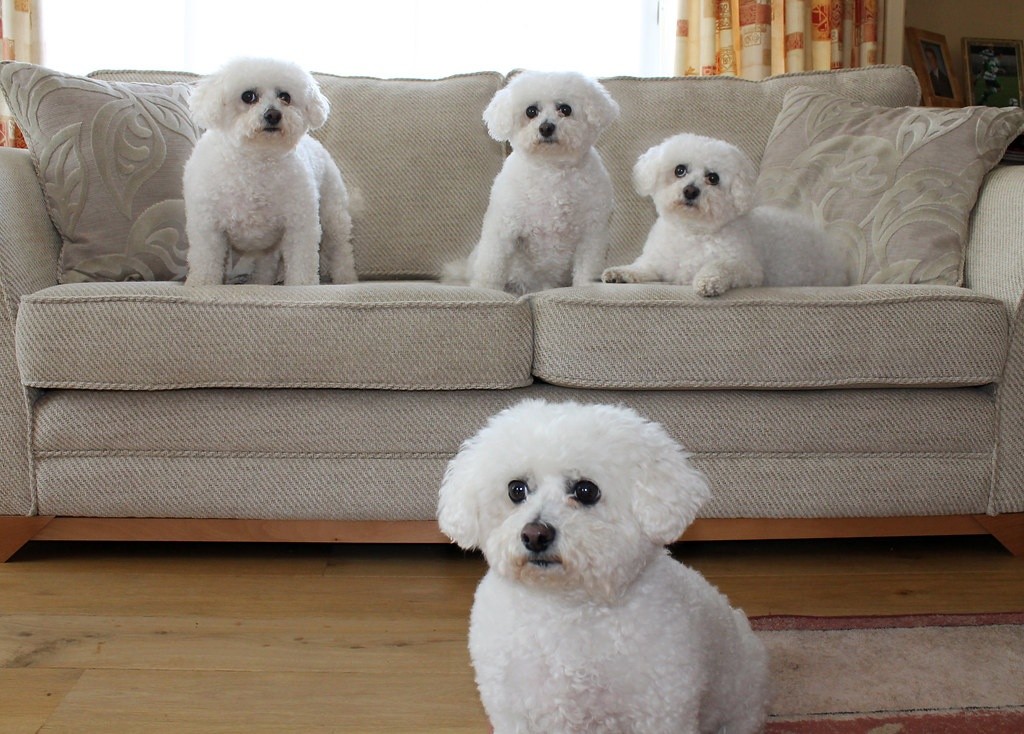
[0,147,1024,561]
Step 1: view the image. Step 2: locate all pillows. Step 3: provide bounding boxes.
[753,86,1024,288]
[0,60,255,284]
[85,67,506,276]
[591,63,921,279]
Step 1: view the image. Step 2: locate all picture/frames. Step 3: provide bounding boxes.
[905,26,965,107]
[959,36,1024,111]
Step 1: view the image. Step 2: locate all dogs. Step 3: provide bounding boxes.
[438,69,620,292]
[600,132,850,297]
[181,55,360,287]
[435,396,771,734]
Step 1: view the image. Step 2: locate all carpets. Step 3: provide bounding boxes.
[751,610,1024,734]
